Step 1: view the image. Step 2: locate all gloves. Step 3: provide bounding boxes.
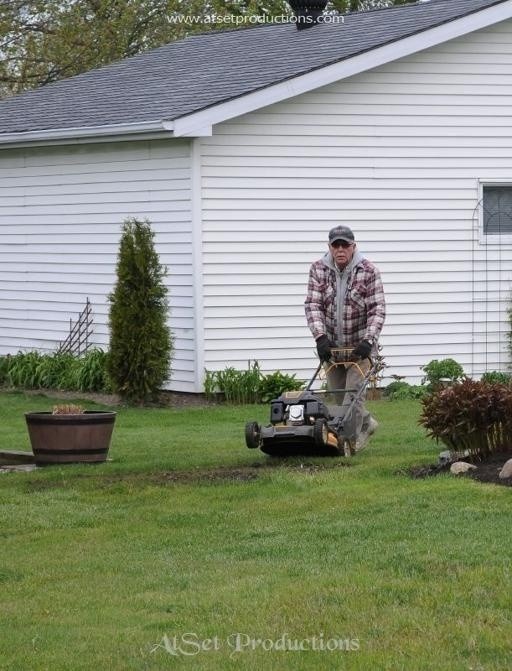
[316,335,335,362]
[351,340,372,362]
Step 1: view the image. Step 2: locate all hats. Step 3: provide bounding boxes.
[327,225,354,244]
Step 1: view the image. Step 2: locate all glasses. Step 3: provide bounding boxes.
[330,242,352,250]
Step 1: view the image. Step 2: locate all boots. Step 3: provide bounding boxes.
[354,413,378,450]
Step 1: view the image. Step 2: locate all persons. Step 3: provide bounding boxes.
[304,226,385,452]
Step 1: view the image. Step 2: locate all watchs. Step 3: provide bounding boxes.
[364,338,373,347]
